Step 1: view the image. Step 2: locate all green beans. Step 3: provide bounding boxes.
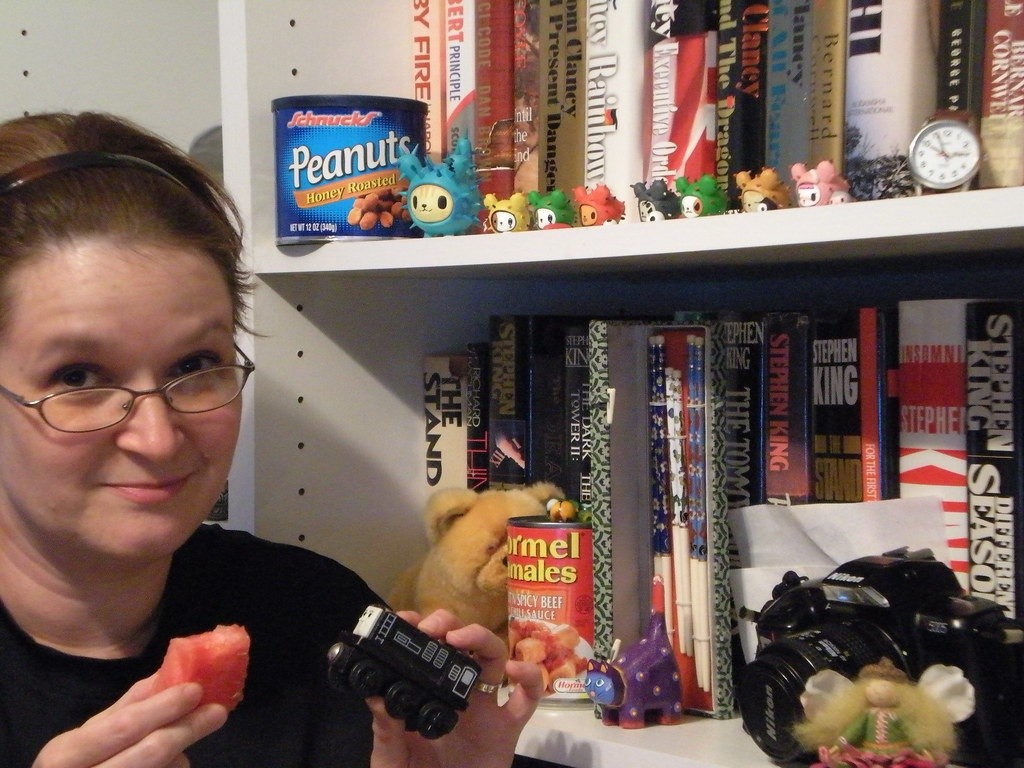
[348,189,411,229]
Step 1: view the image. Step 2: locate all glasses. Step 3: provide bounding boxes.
[0,342,256,433]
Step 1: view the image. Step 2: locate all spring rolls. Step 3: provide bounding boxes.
[509,621,581,695]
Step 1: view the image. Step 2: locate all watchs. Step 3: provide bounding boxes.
[909,111,983,197]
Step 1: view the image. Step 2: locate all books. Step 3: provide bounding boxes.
[412,0,1024,237]
[425,296,1024,719]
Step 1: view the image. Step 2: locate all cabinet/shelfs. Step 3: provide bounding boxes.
[215,0,1024,768]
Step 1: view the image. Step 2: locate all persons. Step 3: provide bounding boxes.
[0,110,544,768]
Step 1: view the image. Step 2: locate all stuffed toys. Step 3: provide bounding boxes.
[384,483,568,653]
